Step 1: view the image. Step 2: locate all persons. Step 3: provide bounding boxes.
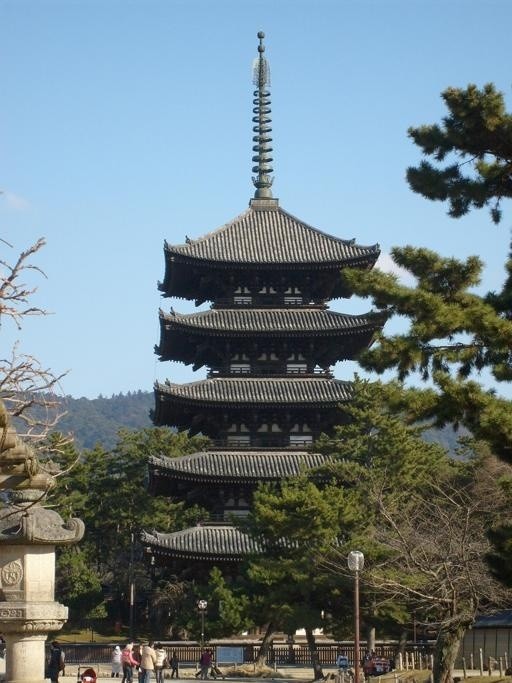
[111,639,179,683]
[360,650,395,679]
[48,641,61,683]
[195,648,214,680]
[0,634,6,650]
[336,649,349,673]
[59,648,66,676]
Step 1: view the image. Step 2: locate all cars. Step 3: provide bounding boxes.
[367,654,396,676]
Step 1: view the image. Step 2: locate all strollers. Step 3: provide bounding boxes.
[76,666,98,683]
[211,663,225,679]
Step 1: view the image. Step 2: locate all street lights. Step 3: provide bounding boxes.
[348,551,365,683]
